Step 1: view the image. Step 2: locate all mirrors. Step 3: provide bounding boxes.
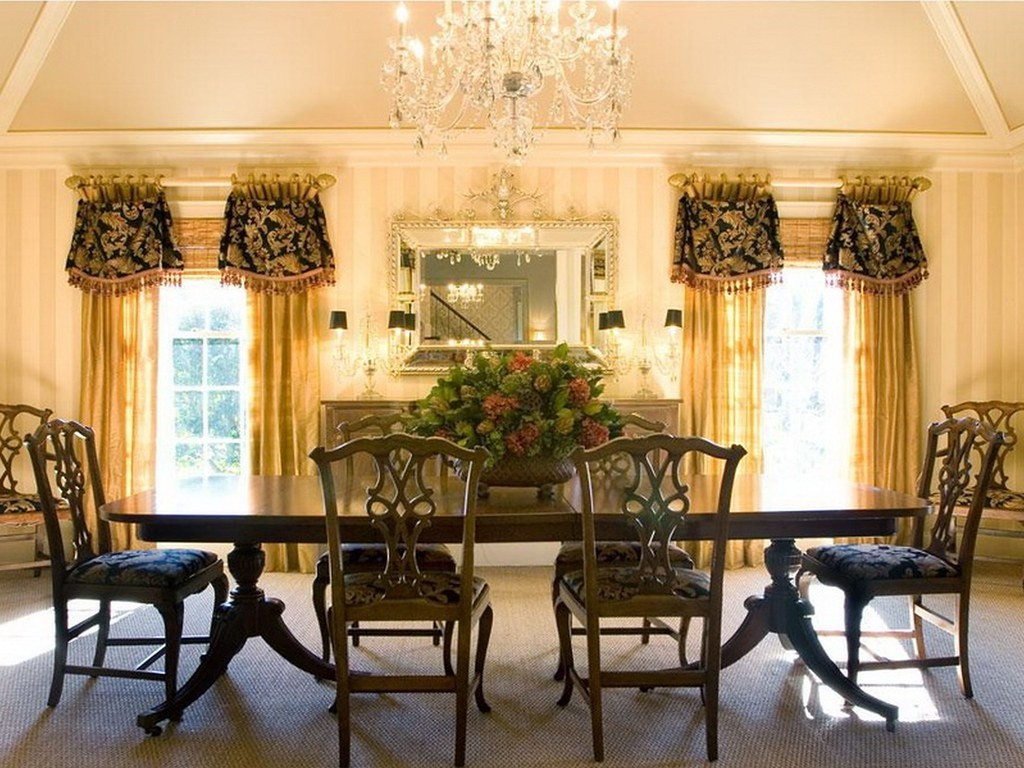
[385,167,620,379]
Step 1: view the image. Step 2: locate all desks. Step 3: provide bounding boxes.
[97,476,931,735]
[320,400,685,547]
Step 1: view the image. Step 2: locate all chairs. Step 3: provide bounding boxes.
[551,433,749,760]
[310,430,497,768]
[311,407,455,677]
[0,403,80,572]
[26,418,230,722]
[927,399,1024,566]
[799,416,1006,710]
[552,413,697,685]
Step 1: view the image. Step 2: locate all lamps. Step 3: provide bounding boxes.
[378,0,636,167]
[447,282,485,310]
[534,322,547,331]
[325,308,417,401]
[598,309,685,398]
